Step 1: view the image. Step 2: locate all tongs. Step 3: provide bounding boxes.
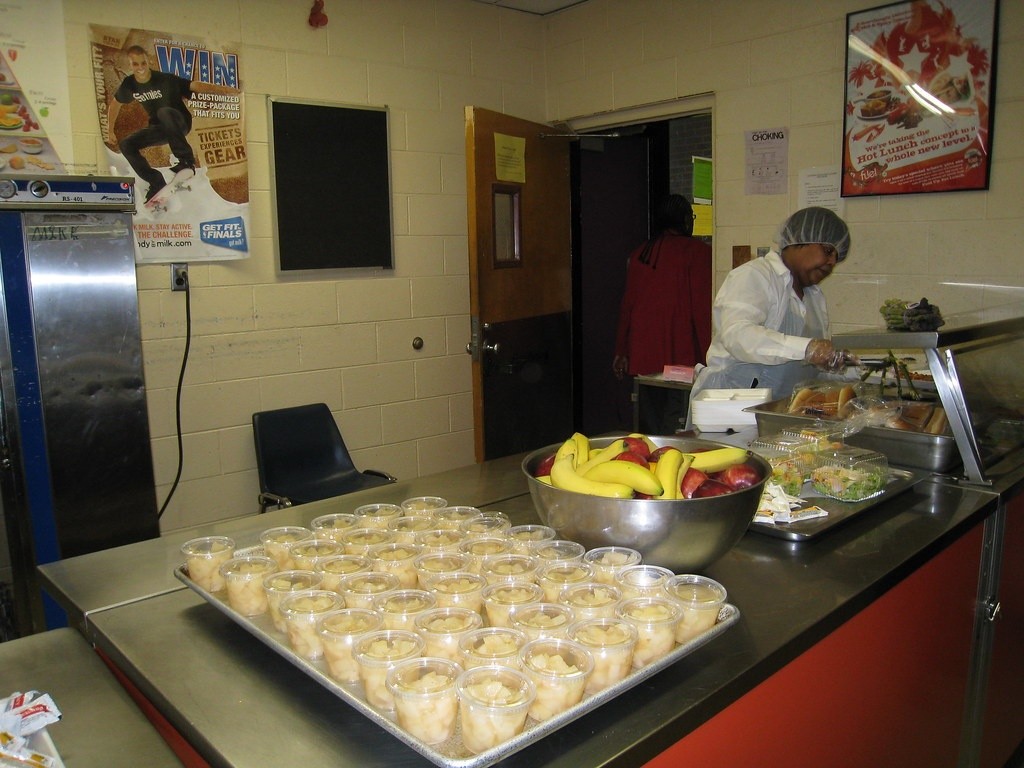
[841,358,891,368]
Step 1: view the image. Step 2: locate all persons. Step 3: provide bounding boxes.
[612,194,712,397]
[683,206,866,432]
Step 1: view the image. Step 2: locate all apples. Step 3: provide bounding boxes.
[537,438,763,499]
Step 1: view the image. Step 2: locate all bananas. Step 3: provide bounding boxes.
[534,432,752,499]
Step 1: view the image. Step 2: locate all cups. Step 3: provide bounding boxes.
[180,495,726,757]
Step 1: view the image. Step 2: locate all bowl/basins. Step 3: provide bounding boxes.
[521,436,773,572]
[749,422,889,501]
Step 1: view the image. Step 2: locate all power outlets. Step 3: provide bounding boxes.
[170,262,189,292]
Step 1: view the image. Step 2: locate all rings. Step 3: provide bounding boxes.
[620,368,623,372]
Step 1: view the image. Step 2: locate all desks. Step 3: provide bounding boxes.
[632,371,694,435]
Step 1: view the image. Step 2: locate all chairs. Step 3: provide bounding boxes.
[250,403,397,515]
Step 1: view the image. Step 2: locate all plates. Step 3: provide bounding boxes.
[856,106,899,119]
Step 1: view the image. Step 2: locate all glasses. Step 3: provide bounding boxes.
[689,215,696,219]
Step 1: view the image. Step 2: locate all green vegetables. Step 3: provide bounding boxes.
[770,466,883,499]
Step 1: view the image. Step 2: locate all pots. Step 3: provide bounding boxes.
[853,90,892,106]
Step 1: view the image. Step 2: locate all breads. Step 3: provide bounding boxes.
[789,386,947,435]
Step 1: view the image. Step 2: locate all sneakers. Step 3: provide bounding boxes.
[169,160,195,173]
[146,177,167,199]
